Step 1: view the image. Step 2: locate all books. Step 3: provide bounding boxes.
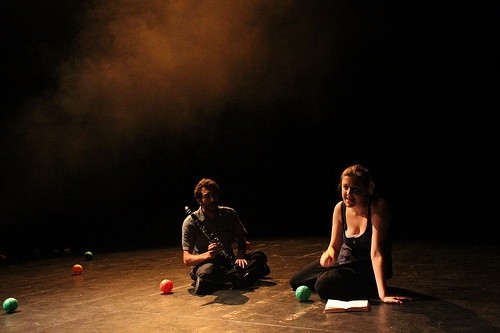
[324,296,370,313]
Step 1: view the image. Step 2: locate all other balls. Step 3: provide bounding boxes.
[85,252,93,259]
[295,285,311,300]
[73,264,83,274]
[160,280,173,292]
[3,298,18,311]
[245,241,250,248]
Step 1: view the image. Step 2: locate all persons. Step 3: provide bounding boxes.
[181,177,270,296]
[289,165,412,305]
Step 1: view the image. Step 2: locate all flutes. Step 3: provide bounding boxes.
[185,206,236,269]
